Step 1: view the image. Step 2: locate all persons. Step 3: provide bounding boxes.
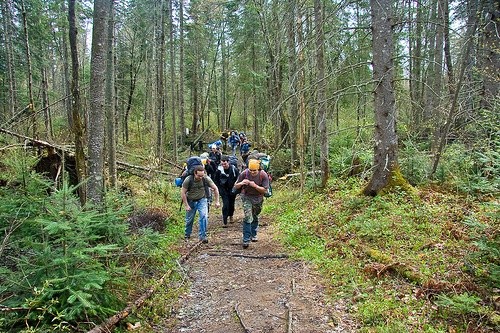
[180,166,219,243]
[234,159,269,248]
[200,130,266,225]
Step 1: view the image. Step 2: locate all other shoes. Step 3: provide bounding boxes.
[251,235,258,241]
[200,237,208,244]
[185,235,190,240]
[222,223,227,228]
[243,241,249,247]
[230,216,234,223]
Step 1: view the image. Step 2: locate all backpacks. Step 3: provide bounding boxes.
[174,156,209,195]
[217,156,238,177]
[209,140,223,151]
[242,152,272,197]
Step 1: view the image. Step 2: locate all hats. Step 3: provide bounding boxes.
[248,159,261,170]
